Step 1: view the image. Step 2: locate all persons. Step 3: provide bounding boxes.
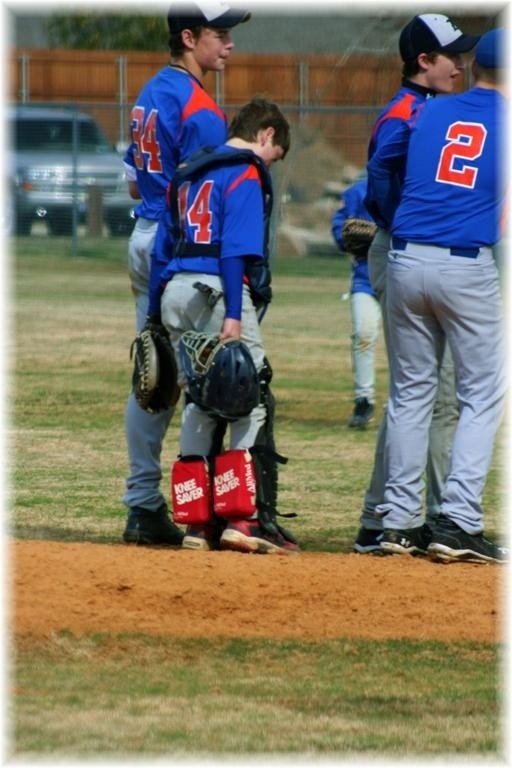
[368,27,508,564]
[331,180,384,427]
[148,99,300,552]
[122,6,301,546]
[351,14,481,553]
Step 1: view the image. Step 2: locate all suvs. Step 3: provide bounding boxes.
[5,108,140,232]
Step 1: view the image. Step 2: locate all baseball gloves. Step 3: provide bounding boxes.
[342,218,376,244]
[132,323,179,415]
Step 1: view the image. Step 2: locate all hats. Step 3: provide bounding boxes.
[399,13,501,68]
[168,3,250,34]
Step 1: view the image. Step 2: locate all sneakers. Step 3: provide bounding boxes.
[352,518,507,564]
[124,504,302,553]
[348,396,375,427]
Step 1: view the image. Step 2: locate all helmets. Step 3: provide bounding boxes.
[175,330,260,423]
